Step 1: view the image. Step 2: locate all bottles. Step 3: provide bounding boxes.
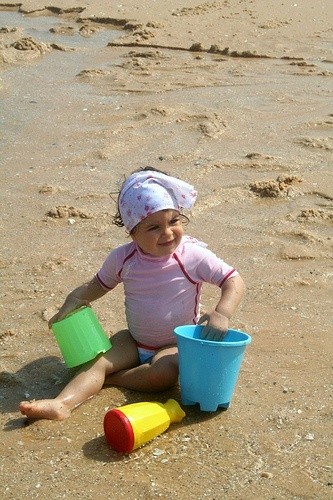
[103,396,186,454]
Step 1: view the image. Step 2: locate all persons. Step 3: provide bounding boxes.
[18,167,245,421]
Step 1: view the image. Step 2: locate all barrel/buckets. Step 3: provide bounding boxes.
[51,305,113,368]
[172,323,252,413]
